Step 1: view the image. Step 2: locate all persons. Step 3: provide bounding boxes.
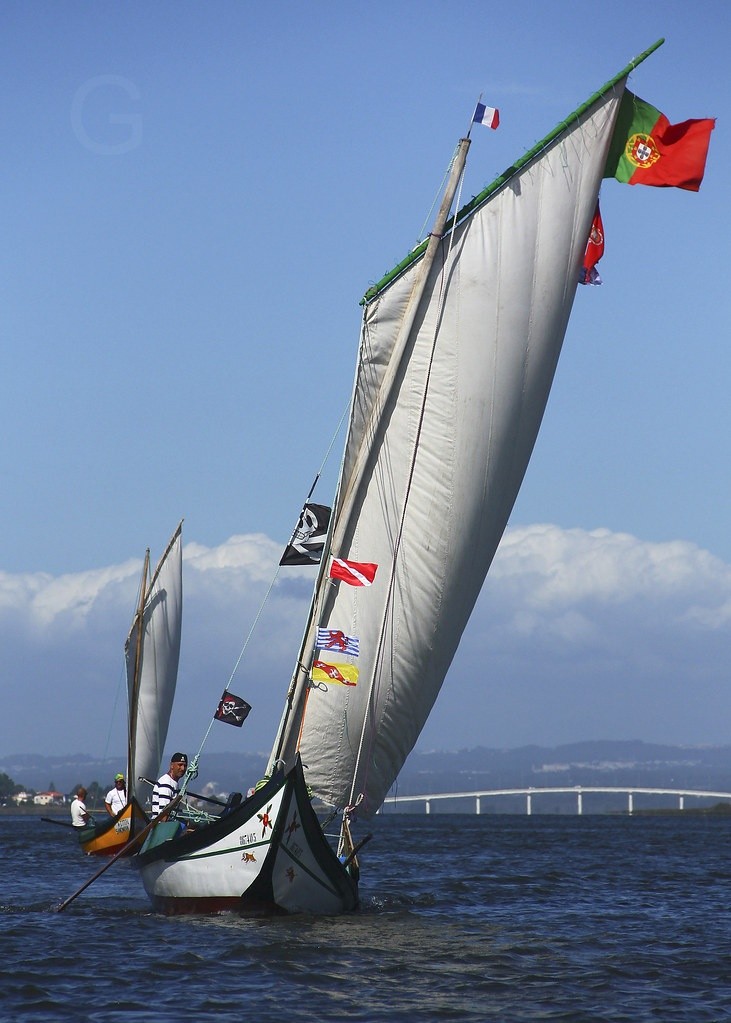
[104,775,127,817]
[70,788,91,831]
[152,753,189,832]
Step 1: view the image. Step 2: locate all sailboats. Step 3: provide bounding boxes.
[69,516,195,854]
[131,34,670,918]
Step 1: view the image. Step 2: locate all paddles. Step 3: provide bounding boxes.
[343,834,372,868]
[51,794,181,915]
[40,817,90,831]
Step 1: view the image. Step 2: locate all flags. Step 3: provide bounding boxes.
[602,87,718,191]
[327,555,379,586]
[214,690,252,727]
[280,502,332,566]
[473,101,500,130]
[309,659,359,686]
[579,196,605,284]
[314,625,360,658]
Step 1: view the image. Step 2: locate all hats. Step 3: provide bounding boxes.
[170,752,187,763]
[114,773,126,784]
